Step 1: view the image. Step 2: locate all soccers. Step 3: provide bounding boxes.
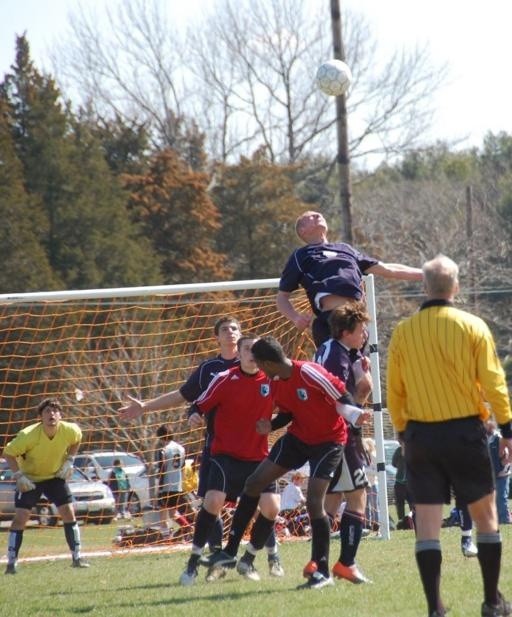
[314,59,352,97]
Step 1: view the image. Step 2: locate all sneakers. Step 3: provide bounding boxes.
[200,552,237,569]
[184,525,193,540]
[481,602,512,617]
[331,561,372,584]
[180,571,197,585]
[4,563,17,574]
[460,535,477,556]
[71,558,89,568]
[268,560,284,576]
[205,565,225,582]
[237,556,260,581]
[297,570,335,590]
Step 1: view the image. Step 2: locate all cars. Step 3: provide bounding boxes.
[123,459,195,517]
[0,459,116,526]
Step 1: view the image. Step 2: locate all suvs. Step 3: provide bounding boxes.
[74,451,145,481]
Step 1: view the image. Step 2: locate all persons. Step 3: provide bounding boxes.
[109,459,131,518]
[303,304,375,587]
[178,334,281,586]
[276,211,422,407]
[2,399,91,574]
[364,438,380,531]
[117,317,284,586]
[391,443,414,521]
[197,337,372,590]
[452,492,478,557]
[487,419,512,525]
[387,253,512,617]
[280,471,306,515]
[156,424,189,535]
